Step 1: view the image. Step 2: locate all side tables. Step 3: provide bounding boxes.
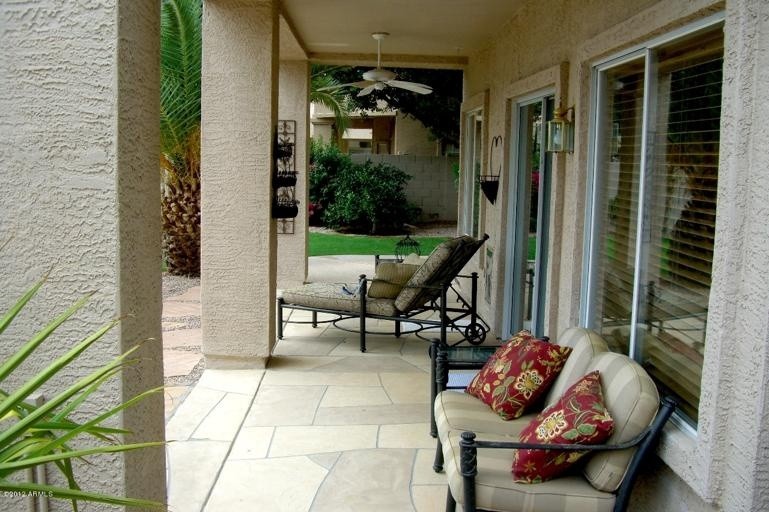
[432,337,502,437]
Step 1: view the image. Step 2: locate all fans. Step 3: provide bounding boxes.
[315,32,434,96]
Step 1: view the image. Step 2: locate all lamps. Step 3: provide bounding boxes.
[547,95,574,154]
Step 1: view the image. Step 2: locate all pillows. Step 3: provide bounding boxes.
[465,329,614,483]
[367,252,424,298]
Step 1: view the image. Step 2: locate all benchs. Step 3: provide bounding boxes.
[433,327,676,511]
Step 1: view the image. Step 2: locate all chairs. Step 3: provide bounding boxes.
[278,233,489,351]
